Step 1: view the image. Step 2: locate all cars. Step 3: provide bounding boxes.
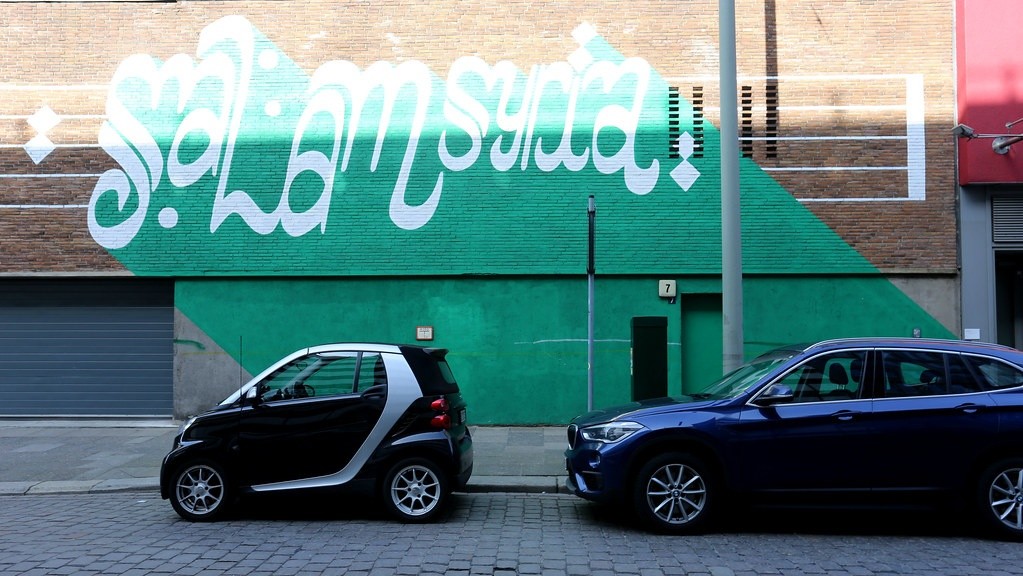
[158,340,475,522]
[559,335,1023,542]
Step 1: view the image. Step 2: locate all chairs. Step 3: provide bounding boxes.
[919,370,942,394]
[826,362,851,401]
[851,359,862,401]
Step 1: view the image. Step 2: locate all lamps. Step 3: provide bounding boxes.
[949,125,1023,154]
